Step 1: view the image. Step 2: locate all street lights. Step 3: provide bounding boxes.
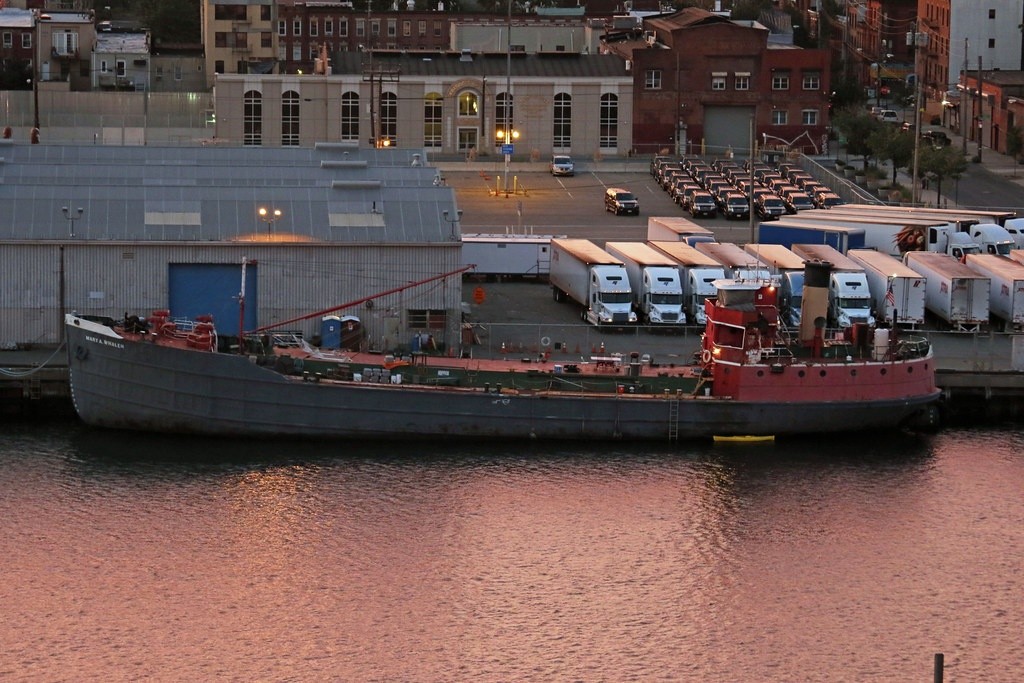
[32,9,52,128]
[497,128,520,191]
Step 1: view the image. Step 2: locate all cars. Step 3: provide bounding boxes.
[872,107,883,114]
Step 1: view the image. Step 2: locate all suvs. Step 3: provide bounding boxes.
[922,130,951,150]
[650,156,842,219]
[550,154,575,176]
[604,188,640,216]
[878,110,898,123]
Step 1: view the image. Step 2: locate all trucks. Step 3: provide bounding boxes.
[965,249,1024,323]
[648,216,716,247]
[650,241,876,328]
[846,248,928,331]
[550,237,638,335]
[903,251,991,331]
[759,203,1024,270]
[605,241,688,334]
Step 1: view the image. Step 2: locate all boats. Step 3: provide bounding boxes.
[64,256,943,439]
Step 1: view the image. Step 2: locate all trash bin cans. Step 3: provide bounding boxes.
[555,364,562,373]
[630,363,641,376]
[624,366,630,375]
[555,342,561,350]
[321,316,361,351]
